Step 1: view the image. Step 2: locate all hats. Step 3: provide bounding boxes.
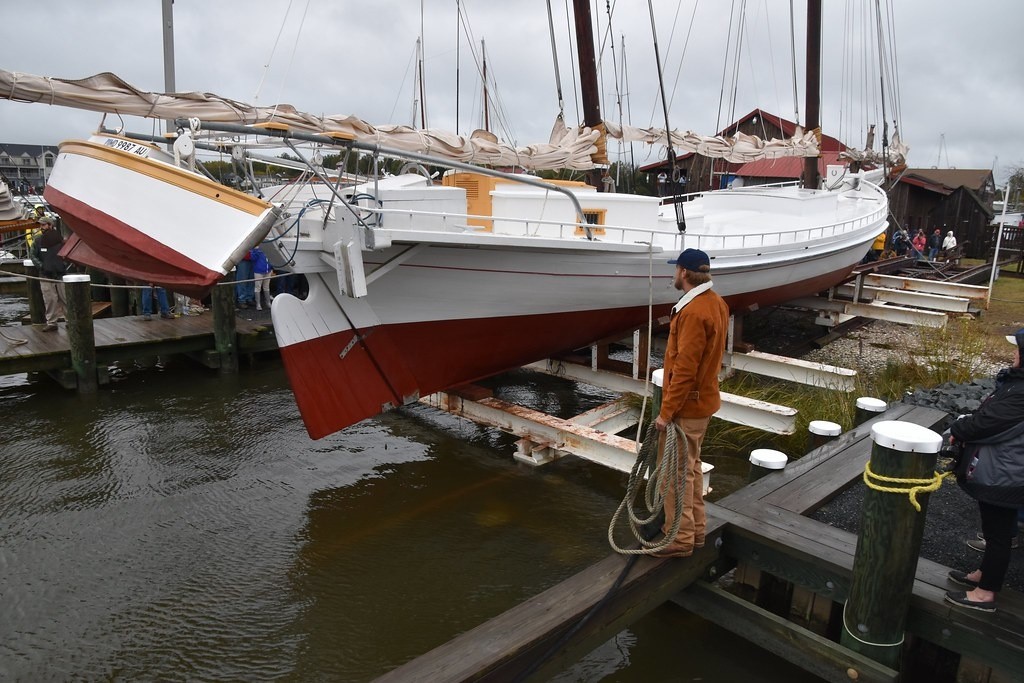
[1006,335,1018,345]
[667,249,710,273]
[36,217,54,225]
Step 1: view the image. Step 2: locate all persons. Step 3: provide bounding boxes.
[871,232,885,256]
[944,329,1024,613]
[680,175,686,194]
[912,229,926,259]
[657,172,667,197]
[926,229,943,261]
[942,231,957,251]
[25,206,47,248]
[641,248,728,559]
[30,215,70,331]
[142,284,176,321]
[236,246,273,311]
[891,224,911,255]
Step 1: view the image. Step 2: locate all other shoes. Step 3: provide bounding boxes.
[977,532,1018,548]
[43,325,58,332]
[945,590,997,612]
[642,541,693,559]
[237,303,249,309]
[663,525,706,546]
[144,315,151,320]
[248,301,256,305]
[948,570,978,588]
[967,540,987,553]
[161,313,175,319]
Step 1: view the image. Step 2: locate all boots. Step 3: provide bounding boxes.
[255,292,263,311]
[264,290,271,309]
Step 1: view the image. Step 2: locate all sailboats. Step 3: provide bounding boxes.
[9,0,909,441]
